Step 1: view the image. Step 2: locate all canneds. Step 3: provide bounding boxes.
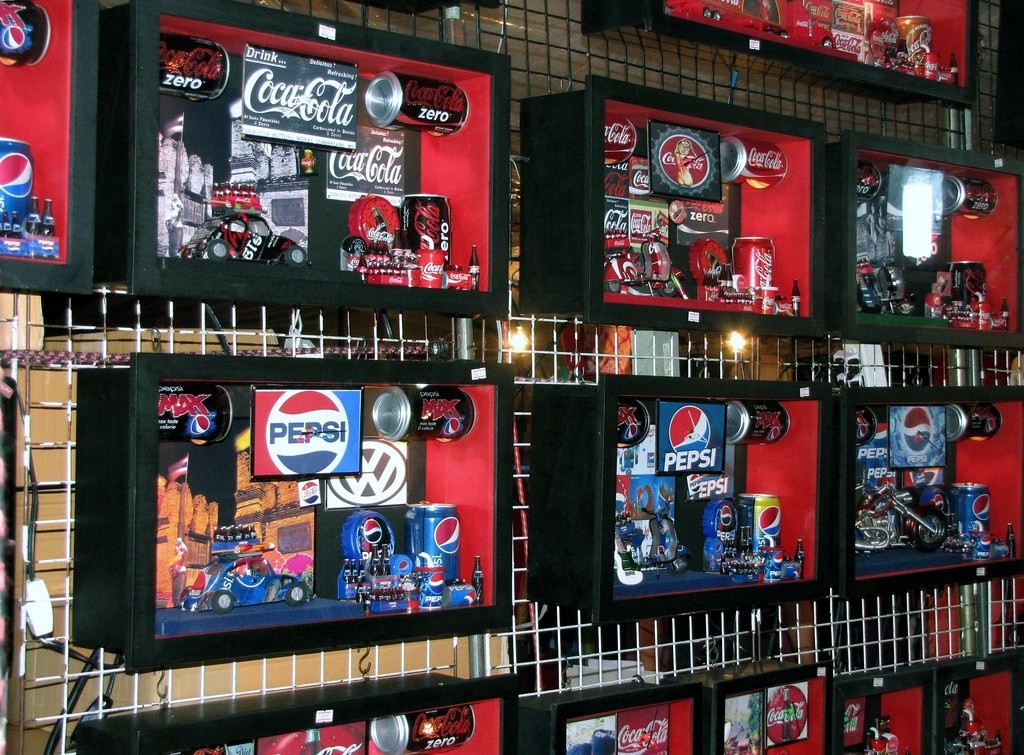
[614,399,650,448]
[398,194,451,269]
[950,482,990,542]
[945,259,986,317]
[370,703,476,754]
[0,0,51,66]
[857,158,882,201]
[404,501,461,585]
[855,405,877,447]
[372,385,477,441]
[0,136,35,233]
[725,401,790,445]
[364,71,470,136]
[945,403,1003,442]
[156,31,229,99]
[717,136,789,190]
[932,175,998,218]
[893,16,932,57]
[731,237,776,296]
[602,113,638,163]
[156,384,233,442]
[736,493,782,555]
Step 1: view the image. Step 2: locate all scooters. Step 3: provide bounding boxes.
[617,498,693,576]
[604,226,684,298]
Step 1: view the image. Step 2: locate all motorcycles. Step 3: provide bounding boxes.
[853,256,915,316]
[854,482,949,556]
[179,543,308,612]
[865,716,911,755]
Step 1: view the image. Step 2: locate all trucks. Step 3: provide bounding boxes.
[661,1,839,53]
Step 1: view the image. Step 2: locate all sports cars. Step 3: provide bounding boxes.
[177,213,309,266]
